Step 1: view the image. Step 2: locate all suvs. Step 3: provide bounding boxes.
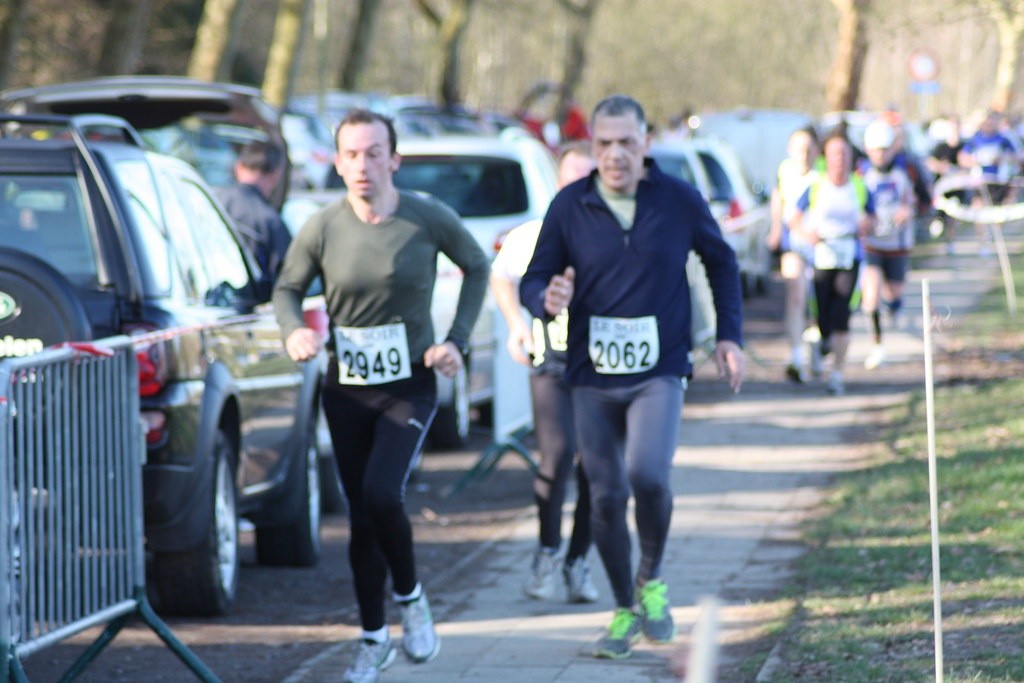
[298,98,518,140]
[288,126,559,420]
[0,113,339,617]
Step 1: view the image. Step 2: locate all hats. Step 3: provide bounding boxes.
[863,121,896,151]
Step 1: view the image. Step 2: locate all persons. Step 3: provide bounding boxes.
[765,126,824,384]
[207,139,292,302]
[857,111,934,373]
[489,140,716,605]
[925,108,1024,257]
[520,95,745,660]
[788,121,878,395]
[272,108,491,683]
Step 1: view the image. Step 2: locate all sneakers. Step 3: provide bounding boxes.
[593,608,644,660]
[786,363,804,383]
[342,627,396,683]
[810,345,826,376]
[823,375,844,396]
[635,578,675,645]
[393,586,440,663]
[564,557,597,604]
[525,546,558,599]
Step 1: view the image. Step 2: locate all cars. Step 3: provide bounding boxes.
[0,73,289,225]
[692,114,921,201]
[654,144,778,304]
